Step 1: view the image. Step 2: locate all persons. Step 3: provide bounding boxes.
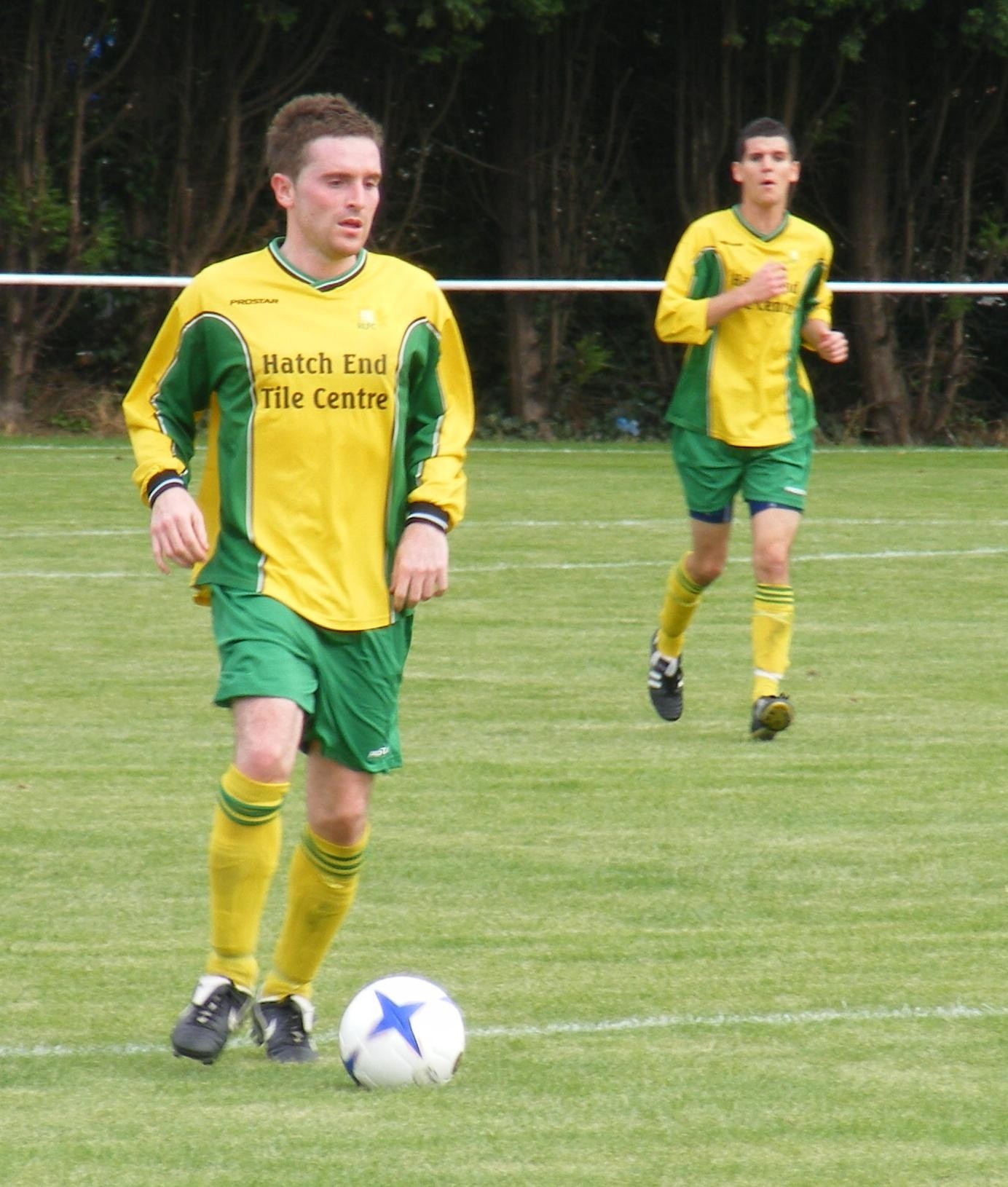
[645,117,850,743]
[116,92,475,1062]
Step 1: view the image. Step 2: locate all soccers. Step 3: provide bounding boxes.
[339,976,467,1089]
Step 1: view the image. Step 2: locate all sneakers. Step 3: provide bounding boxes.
[250,984,318,1063]
[171,973,255,1065]
[648,627,684,721]
[751,692,793,740]
